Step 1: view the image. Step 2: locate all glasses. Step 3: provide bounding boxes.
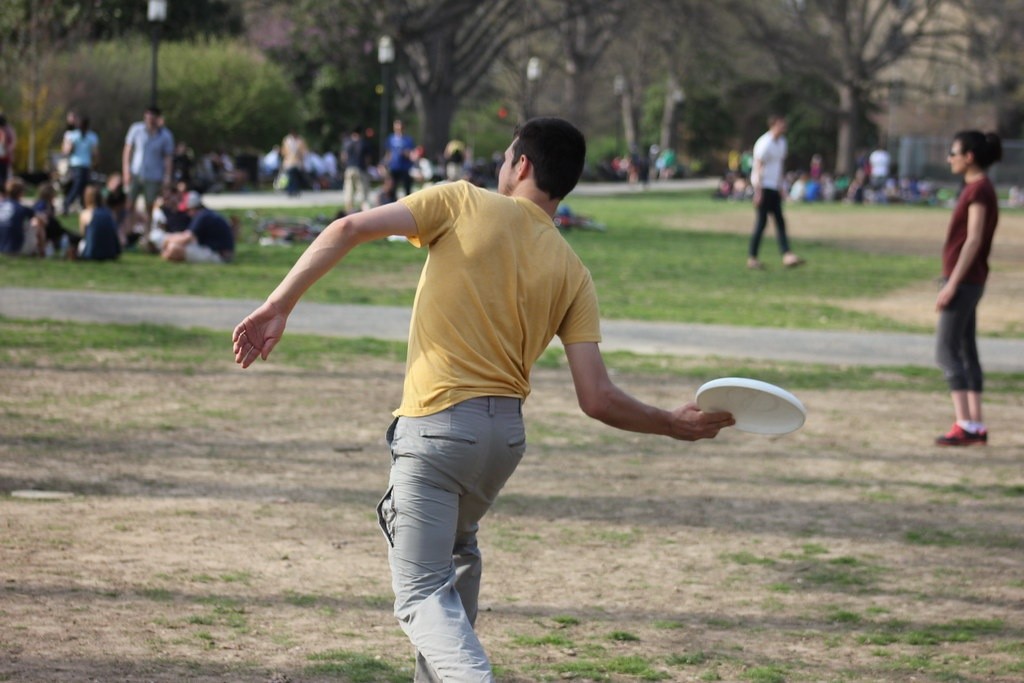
[947,150,965,158]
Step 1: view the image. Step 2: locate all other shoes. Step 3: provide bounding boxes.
[782,254,805,268]
[748,260,765,271]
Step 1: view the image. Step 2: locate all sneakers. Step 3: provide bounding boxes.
[934,422,979,447]
[978,431,988,447]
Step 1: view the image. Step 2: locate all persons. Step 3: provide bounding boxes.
[0,103,1024,266]
[230,114,734,683]
[932,130,1003,445]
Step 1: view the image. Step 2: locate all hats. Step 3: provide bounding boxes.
[178,190,201,212]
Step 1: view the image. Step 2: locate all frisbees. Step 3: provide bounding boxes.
[696,377,808,436]
